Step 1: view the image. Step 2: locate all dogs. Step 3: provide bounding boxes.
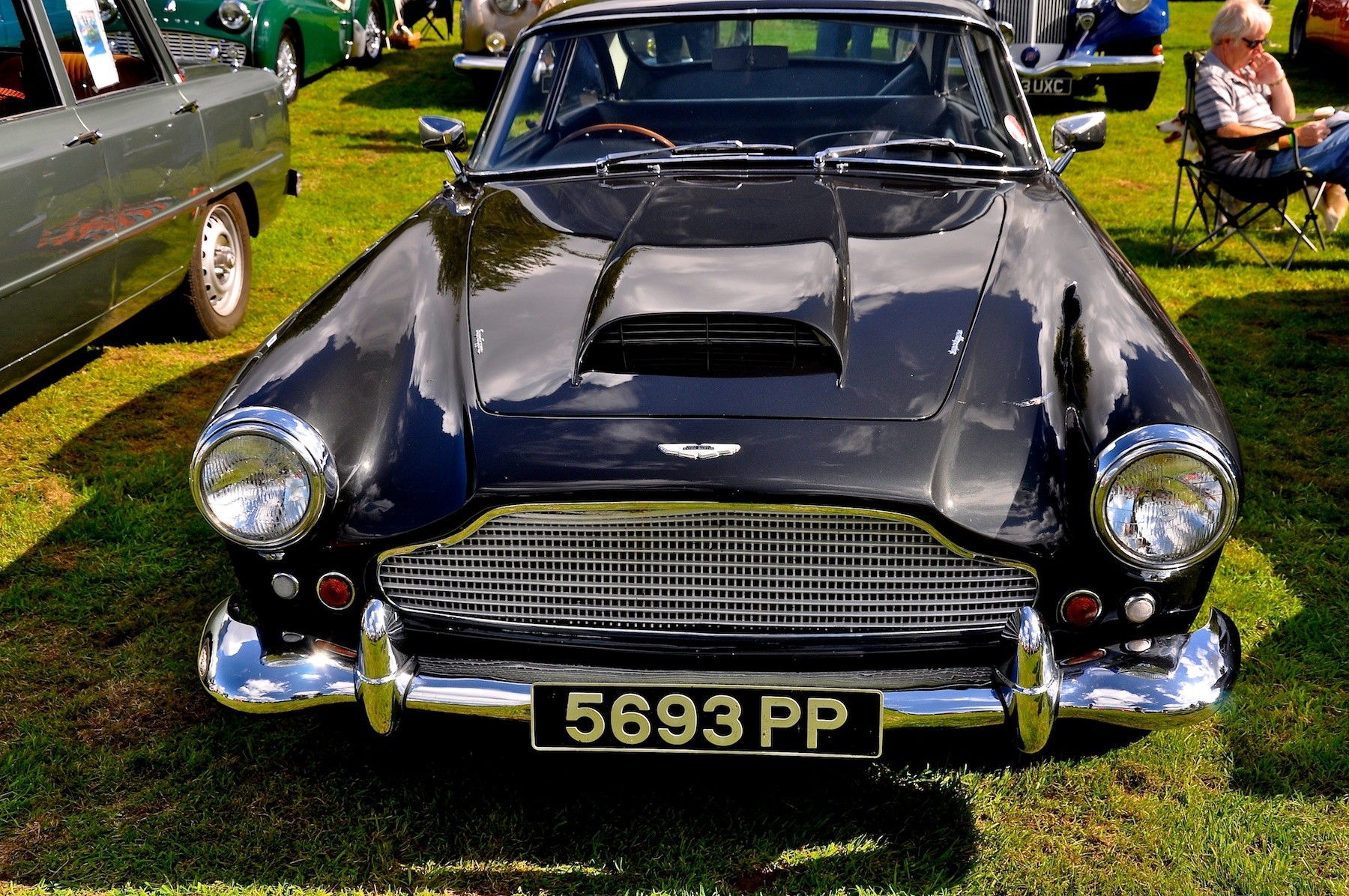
[1313,107,1349,237]
[1155,109,1243,240]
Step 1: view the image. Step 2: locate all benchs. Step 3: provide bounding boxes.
[552,96,954,142]
[0,52,150,100]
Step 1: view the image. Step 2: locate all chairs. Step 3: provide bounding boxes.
[419,0,455,42]
[1167,48,1333,272]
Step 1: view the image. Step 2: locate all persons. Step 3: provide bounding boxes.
[1195,0,1349,233]
[653,21,720,64]
[815,22,874,60]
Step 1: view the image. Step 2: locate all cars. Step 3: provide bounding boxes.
[1,0,401,405]
[886,0,1172,113]
[180,0,1248,768]
[0,0,305,401]
[450,0,695,100]
[1286,0,1349,96]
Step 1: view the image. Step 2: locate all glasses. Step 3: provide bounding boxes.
[1242,37,1267,49]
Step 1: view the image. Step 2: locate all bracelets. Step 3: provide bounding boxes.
[1289,134,1294,148]
[1268,70,1286,86]
